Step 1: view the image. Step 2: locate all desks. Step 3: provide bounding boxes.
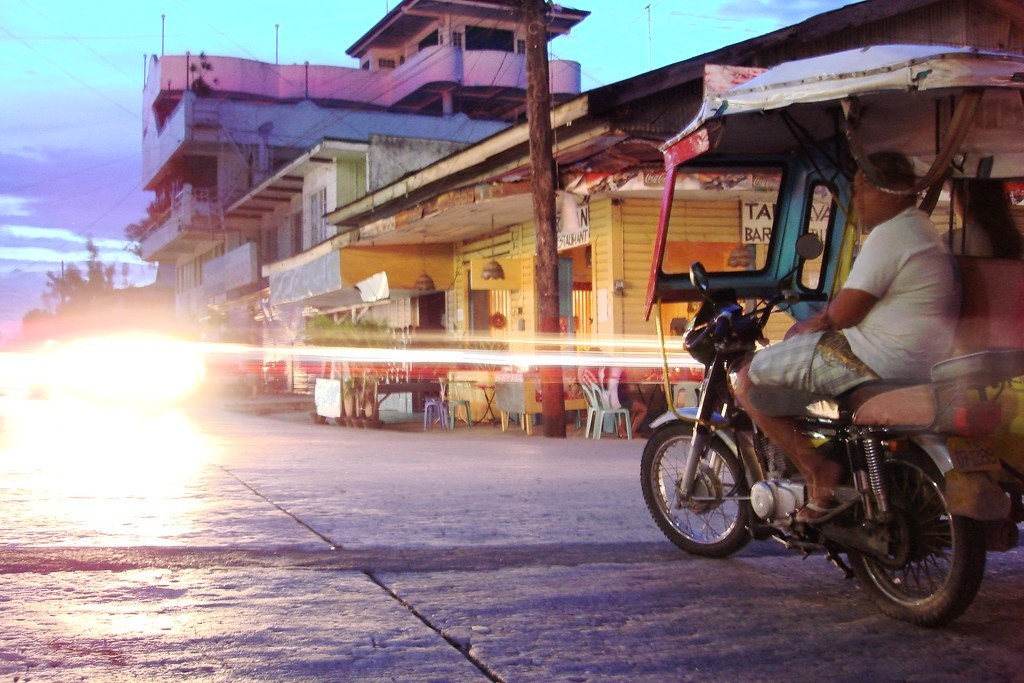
[450,370,523,423]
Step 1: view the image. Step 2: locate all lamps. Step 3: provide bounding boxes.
[414,236,435,291]
[481,216,504,281]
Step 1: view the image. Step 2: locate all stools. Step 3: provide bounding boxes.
[424,402,443,430]
[477,385,496,427]
[450,400,472,429]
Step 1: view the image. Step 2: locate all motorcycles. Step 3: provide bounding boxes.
[639,232,1023,627]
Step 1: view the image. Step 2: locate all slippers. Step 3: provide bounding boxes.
[796,492,861,524]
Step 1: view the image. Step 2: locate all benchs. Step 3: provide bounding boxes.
[495,381,591,436]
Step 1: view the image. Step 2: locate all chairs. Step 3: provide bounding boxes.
[581,384,618,438]
[592,383,632,440]
[664,382,702,424]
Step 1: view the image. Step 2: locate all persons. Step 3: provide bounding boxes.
[668,316,705,383]
[600,365,647,440]
[733,147,967,526]
[577,347,604,408]
[939,173,1024,259]
